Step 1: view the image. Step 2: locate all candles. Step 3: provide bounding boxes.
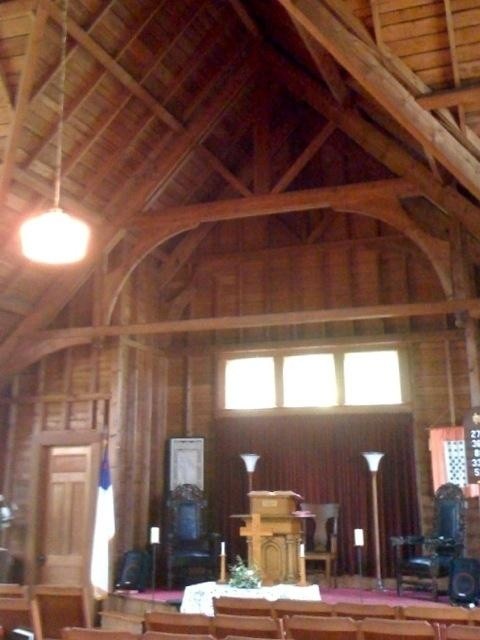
[353,529,365,546]
[300,543,305,556]
[221,541,226,555]
[150,526,160,544]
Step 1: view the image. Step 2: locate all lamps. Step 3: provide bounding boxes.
[240,452,260,493]
[18,0,92,265]
[360,450,390,594]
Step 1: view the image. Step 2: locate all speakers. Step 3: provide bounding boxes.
[449,558,480,603]
[115,550,151,591]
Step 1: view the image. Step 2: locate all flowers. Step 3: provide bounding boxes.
[228,554,264,590]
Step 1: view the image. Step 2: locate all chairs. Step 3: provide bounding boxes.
[298,503,342,589]
[0,582,480,640]
[160,483,223,590]
[389,483,469,602]
